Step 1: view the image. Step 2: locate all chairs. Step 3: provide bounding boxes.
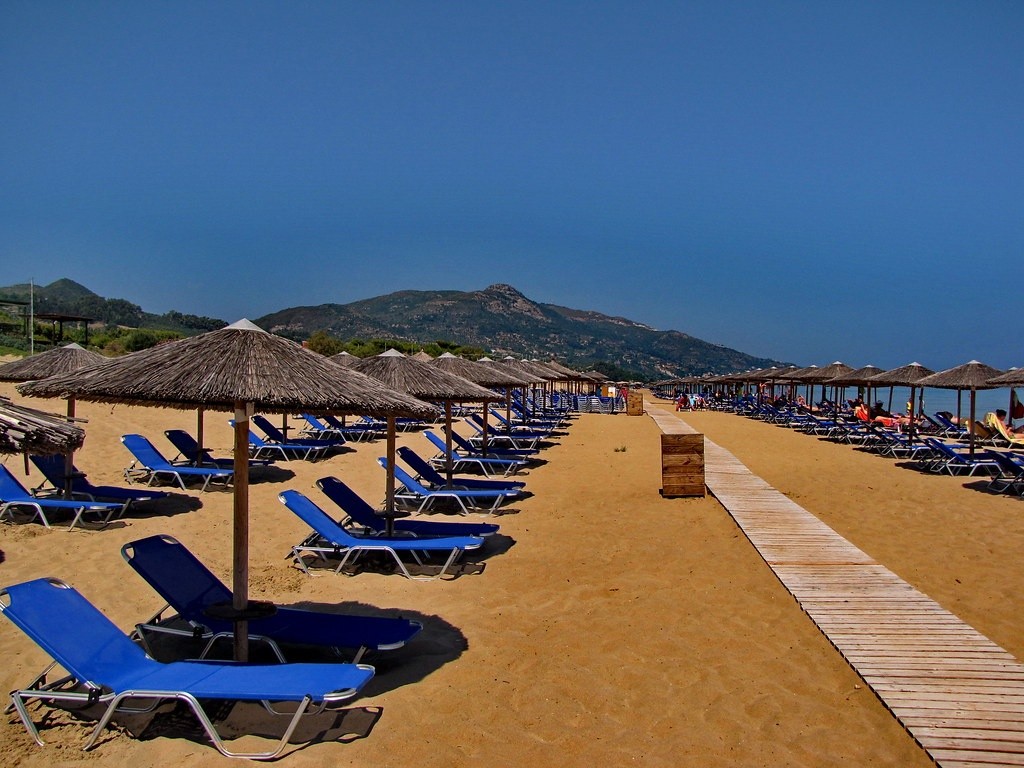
[121,534,423,663]
[651,390,1024,492]
[489,407,557,438]
[469,413,547,445]
[461,416,547,448]
[440,425,540,465]
[396,446,526,503]
[252,415,344,455]
[316,476,500,567]
[0,577,383,760]
[301,414,427,444]
[228,419,329,462]
[376,456,527,518]
[278,489,484,580]
[163,429,275,479]
[423,430,529,478]
[488,388,624,415]
[28,455,172,515]
[119,434,234,493]
[0,463,127,532]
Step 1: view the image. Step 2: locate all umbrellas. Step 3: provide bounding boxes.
[648,361,1024,465]
[0,318,609,665]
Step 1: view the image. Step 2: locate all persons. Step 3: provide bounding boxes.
[672,383,807,412]
[842,394,1024,439]
